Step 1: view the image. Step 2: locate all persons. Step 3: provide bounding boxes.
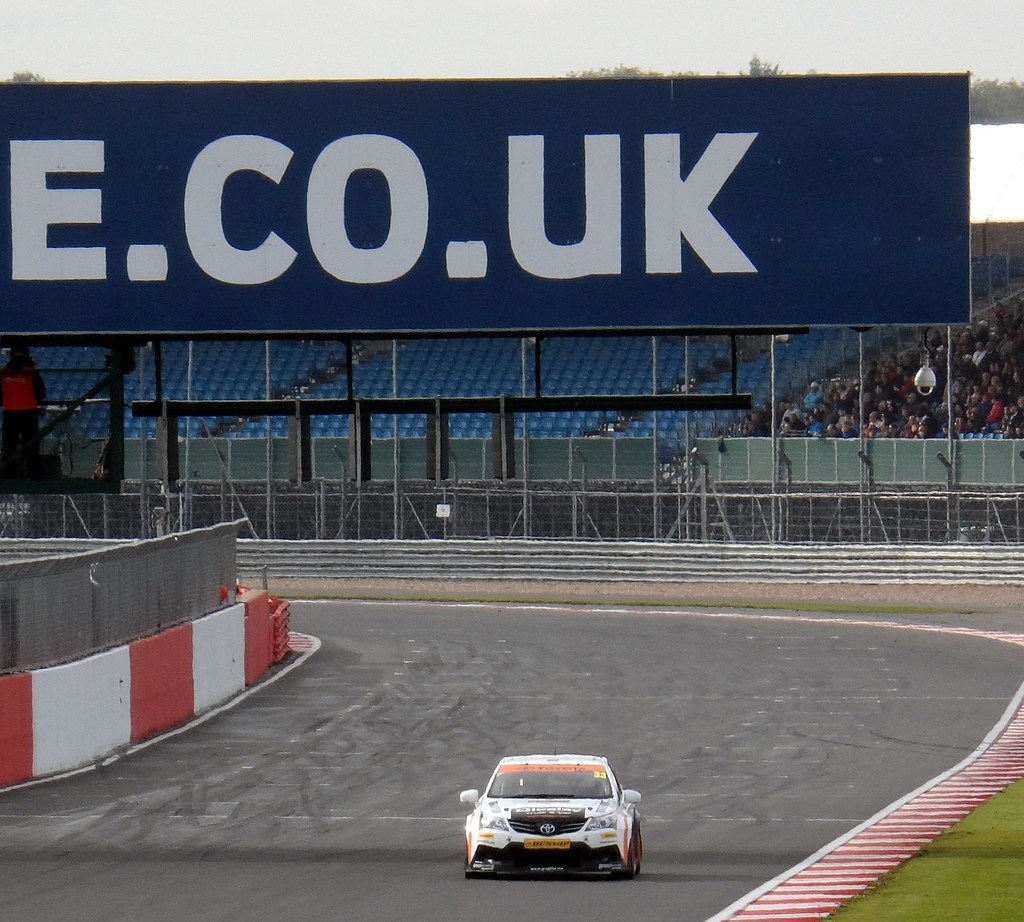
[147,503,184,540]
[737,293,1024,442]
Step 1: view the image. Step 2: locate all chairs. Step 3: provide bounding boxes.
[1,252,1024,439]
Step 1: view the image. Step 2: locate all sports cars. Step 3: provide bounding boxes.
[459,754,643,880]
